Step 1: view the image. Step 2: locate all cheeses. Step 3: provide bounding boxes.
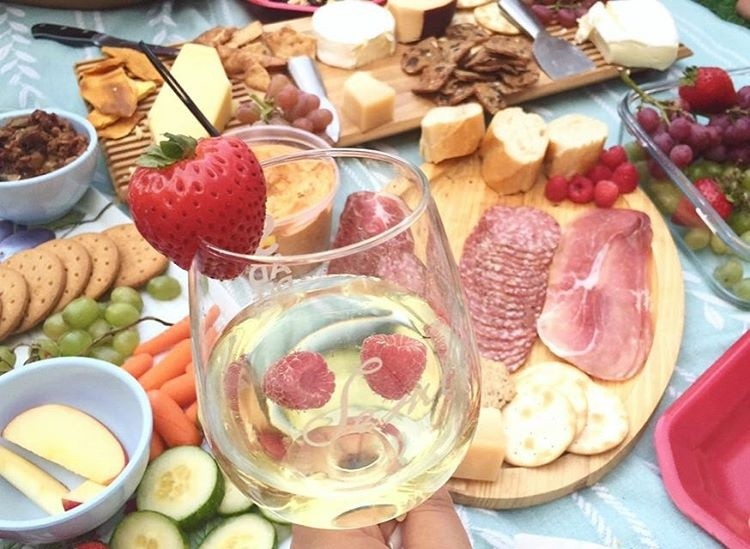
[342,71,396,131]
[389,0,459,44]
[145,43,234,141]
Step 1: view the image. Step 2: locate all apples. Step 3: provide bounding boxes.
[0,402,129,514]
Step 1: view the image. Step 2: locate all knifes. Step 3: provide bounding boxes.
[31,22,181,58]
[287,55,340,144]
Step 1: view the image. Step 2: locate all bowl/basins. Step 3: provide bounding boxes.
[0,108,98,226]
[221,124,341,282]
[617,66,750,312]
[0,355,154,546]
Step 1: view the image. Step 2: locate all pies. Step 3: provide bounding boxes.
[195,23,318,92]
[79,45,165,142]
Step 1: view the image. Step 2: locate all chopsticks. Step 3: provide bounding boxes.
[138,40,221,138]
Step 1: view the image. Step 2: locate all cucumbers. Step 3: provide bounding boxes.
[105,444,278,548]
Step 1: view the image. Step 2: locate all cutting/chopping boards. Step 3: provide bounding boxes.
[77,0,693,199]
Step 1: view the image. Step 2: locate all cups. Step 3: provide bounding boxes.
[188,146,482,532]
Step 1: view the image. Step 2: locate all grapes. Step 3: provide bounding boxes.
[622,82,750,301]
[0,273,182,377]
[233,71,333,139]
[520,0,606,28]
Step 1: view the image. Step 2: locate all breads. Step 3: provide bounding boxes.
[401,24,543,115]
[420,103,609,195]
[452,405,507,482]
[573,0,678,71]
[311,0,396,69]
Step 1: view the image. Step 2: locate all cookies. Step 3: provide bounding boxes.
[452,0,526,35]
[0,222,170,341]
[477,353,629,467]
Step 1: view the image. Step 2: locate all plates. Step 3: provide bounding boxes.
[1,186,242,375]
[654,328,750,549]
[247,0,390,16]
[380,157,687,511]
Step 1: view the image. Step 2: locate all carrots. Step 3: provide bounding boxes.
[119,303,225,457]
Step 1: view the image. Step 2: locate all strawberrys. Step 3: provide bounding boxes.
[679,65,738,113]
[125,134,266,279]
[670,177,741,229]
